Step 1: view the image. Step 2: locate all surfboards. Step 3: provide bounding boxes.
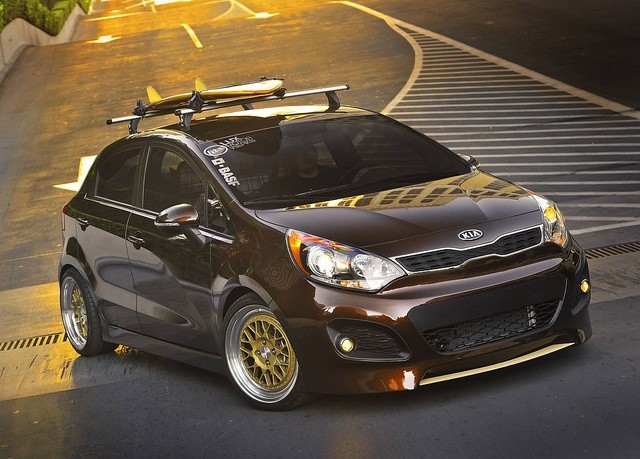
[133,76,284,110]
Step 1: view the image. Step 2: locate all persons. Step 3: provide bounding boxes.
[287,144,353,194]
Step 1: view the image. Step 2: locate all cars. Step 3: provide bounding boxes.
[58,76,593,411]
